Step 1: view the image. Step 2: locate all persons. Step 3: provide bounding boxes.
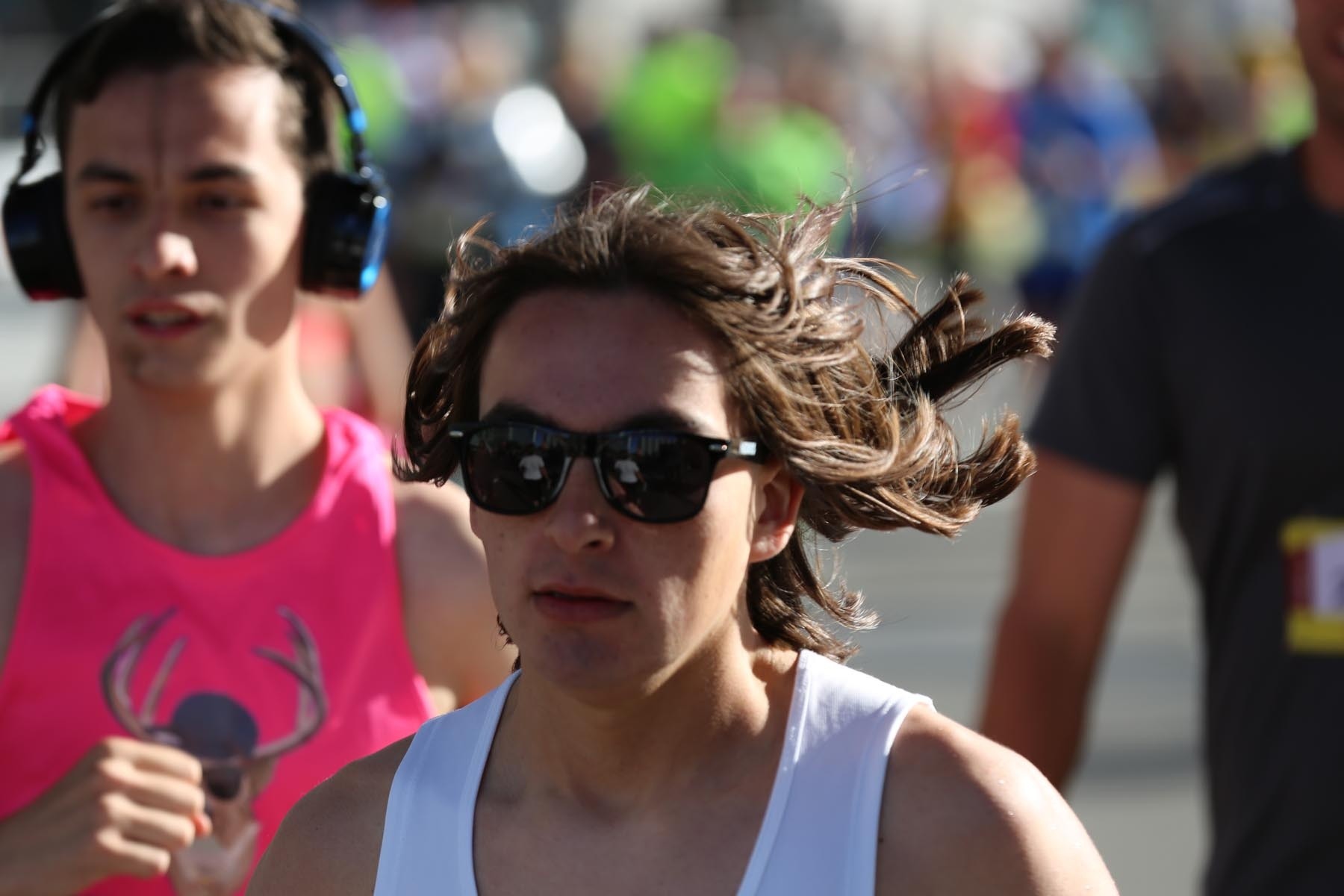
[606,442,652,516]
[0,0,519,895]
[59,3,1320,484]
[973,0,1344,895]
[510,435,555,509]
[236,156,1120,896]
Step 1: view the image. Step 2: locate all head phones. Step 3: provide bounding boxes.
[1,0,390,300]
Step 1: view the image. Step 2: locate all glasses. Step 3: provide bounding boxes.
[444,405,769,524]
